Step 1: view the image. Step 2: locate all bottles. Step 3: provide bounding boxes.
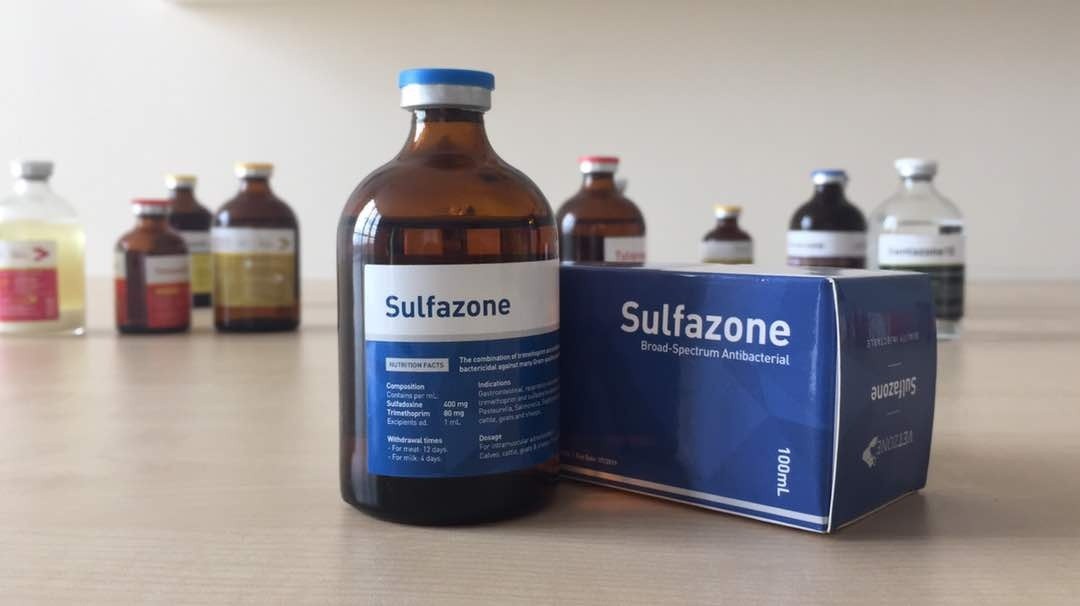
[554,155,646,269]
[868,156,965,337]
[211,162,302,331]
[783,168,868,274]
[170,176,215,306]
[111,199,194,332]
[0,158,87,336]
[698,204,756,265]
[336,68,563,528]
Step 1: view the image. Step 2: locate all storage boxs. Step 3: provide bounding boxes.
[557,263,938,536]
